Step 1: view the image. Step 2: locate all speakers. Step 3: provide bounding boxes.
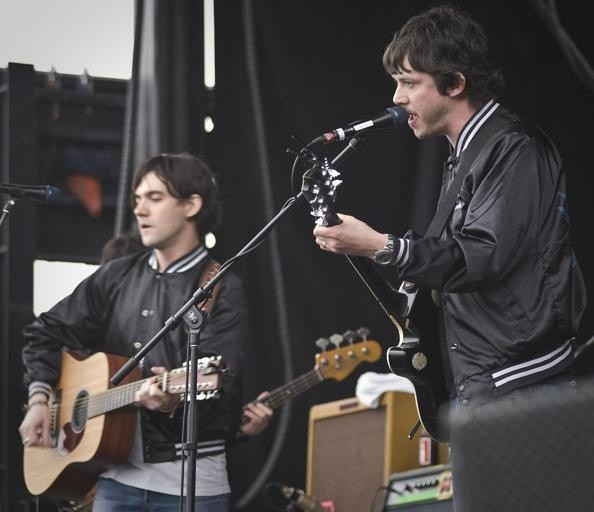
[306,391,448,511]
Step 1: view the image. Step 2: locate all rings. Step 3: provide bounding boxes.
[22,437,30,447]
[320,241,328,248]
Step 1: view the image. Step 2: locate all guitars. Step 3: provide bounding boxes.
[21,347,230,500]
[239,328,383,424]
[301,166,451,443]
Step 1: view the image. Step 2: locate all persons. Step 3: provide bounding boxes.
[310,5,594,414]
[19,151,250,512]
[96,229,276,451]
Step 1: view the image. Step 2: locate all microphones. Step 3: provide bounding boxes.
[0,180,62,205]
[332,104,409,142]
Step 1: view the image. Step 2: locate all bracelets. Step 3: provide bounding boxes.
[23,401,50,412]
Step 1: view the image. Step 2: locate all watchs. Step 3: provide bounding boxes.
[369,232,396,267]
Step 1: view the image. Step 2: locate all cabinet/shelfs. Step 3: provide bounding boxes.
[2,62,129,512]
